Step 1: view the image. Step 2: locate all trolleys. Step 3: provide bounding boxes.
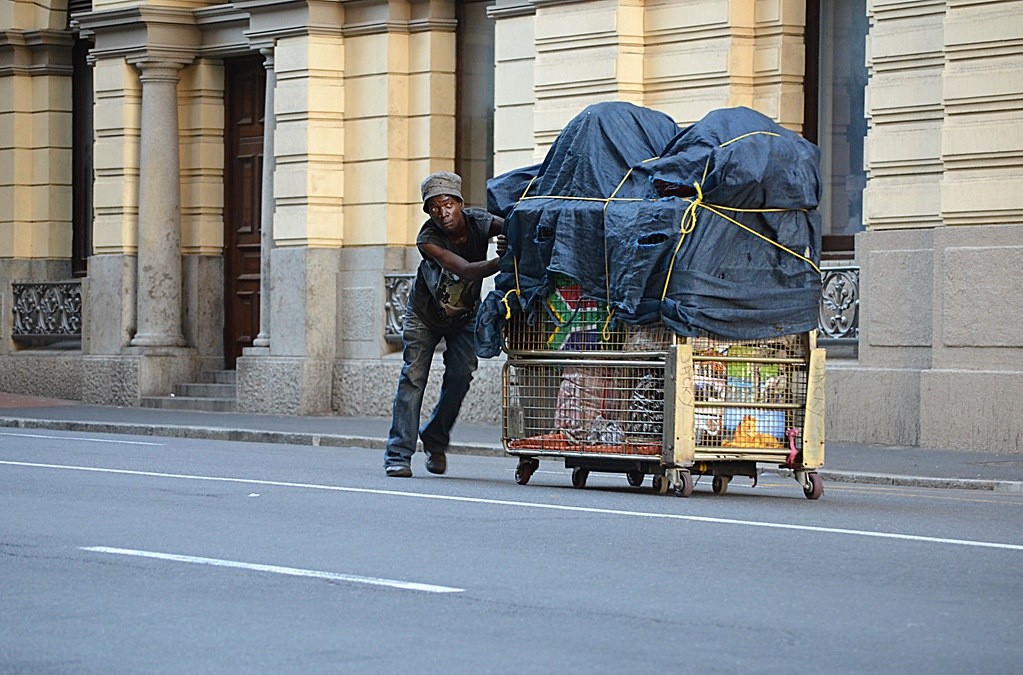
[495,206,829,503]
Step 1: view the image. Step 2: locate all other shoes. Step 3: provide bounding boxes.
[386,465,412,477]
[424,448,447,474]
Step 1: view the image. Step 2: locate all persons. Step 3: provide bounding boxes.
[383,170,509,477]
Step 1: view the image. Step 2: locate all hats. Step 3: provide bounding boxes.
[420,172,464,213]
